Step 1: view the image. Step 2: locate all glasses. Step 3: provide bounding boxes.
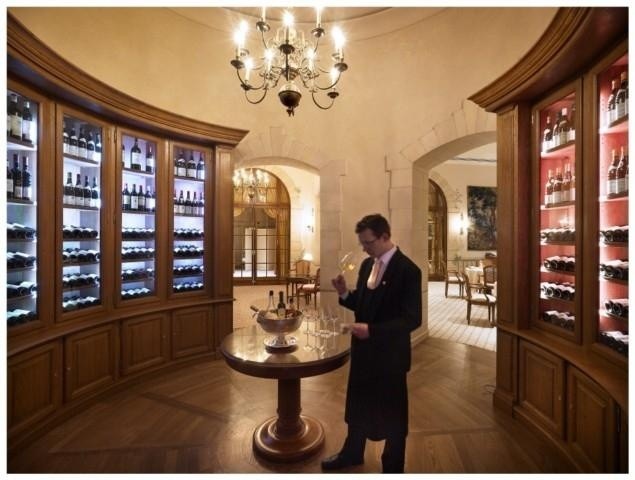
[359,237,377,246]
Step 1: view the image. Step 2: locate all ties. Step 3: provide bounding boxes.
[370,260,380,288]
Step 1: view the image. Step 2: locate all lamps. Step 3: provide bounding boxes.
[229,7,349,119]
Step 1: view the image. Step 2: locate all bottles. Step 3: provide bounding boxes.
[63,224,99,241]
[11,153,23,200]
[542,309,575,333]
[65,172,76,206]
[61,247,101,263]
[123,184,131,210]
[174,245,205,257]
[173,189,179,214]
[7,222,36,241]
[602,297,629,319]
[145,147,155,174]
[138,185,145,212]
[624,85,629,117]
[552,112,562,147]
[11,92,23,142]
[615,72,626,121]
[624,147,629,198]
[122,246,156,260]
[570,163,575,205]
[173,264,205,274]
[63,120,70,155]
[560,163,572,206]
[600,257,628,281]
[543,116,553,152]
[145,186,153,213]
[178,149,187,178]
[539,280,576,303]
[542,255,577,274]
[122,227,155,240]
[91,177,99,207]
[187,150,198,179]
[7,159,15,199]
[6,280,37,298]
[130,185,139,211]
[192,193,200,215]
[278,290,286,320]
[597,331,628,356]
[23,101,33,143]
[267,289,276,313]
[153,191,156,214]
[553,168,562,207]
[84,176,93,207]
[63,272,101,286]
[615,145,627,197]
[607,79,619,126]
[544,169,555,208]
[76,174,84,206]
[173,228,205,240]
[186,191,192,215]
[197,152,206,181]
[566,104,576,142]
[173,280,204,294]
[540,224,576,243]
[120,286,152,300]
[78,128,87,159]
[62,294,101,312]
[87,131,96,161]
[600,224,629,244]
[286,298,298,320]
[607,148,620,199]
[558,108,569,145]
[121,140,125,167]
[199,192,206,216]
[7,251,35,269]
[70,125,78,156]
[174,155,178,175]
[6,306,36,327]
[120,268,155,280]
[23,156,32,201]
[96,134,101,164]
[251,304,277,320]
[179,191,185,216]
[131,138,142,171]
[8,89,12,137]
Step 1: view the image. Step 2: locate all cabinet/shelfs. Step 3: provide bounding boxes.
[115,125,216,378]
[517,42,628,473]
[7,80,115,460]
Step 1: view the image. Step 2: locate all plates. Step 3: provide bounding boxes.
[263,335,297,348]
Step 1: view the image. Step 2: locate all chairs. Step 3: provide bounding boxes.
[461,272,497,327]
[286,260,312,305]
[441,260,465,299]
[483,265,497,286]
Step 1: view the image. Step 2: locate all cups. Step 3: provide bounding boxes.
[312,303,322,337]
[322,304,330,334]
[329,304,340,336]
[304,306,313,334]
[337,251,360,275]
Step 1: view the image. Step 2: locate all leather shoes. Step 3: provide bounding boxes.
[321,452,365,469]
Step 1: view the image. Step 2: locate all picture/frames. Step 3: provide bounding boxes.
[467,186,497,251]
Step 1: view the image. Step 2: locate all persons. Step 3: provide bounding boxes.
[321,214,423,474]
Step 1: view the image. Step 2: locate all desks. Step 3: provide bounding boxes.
[220,317,351,464]
